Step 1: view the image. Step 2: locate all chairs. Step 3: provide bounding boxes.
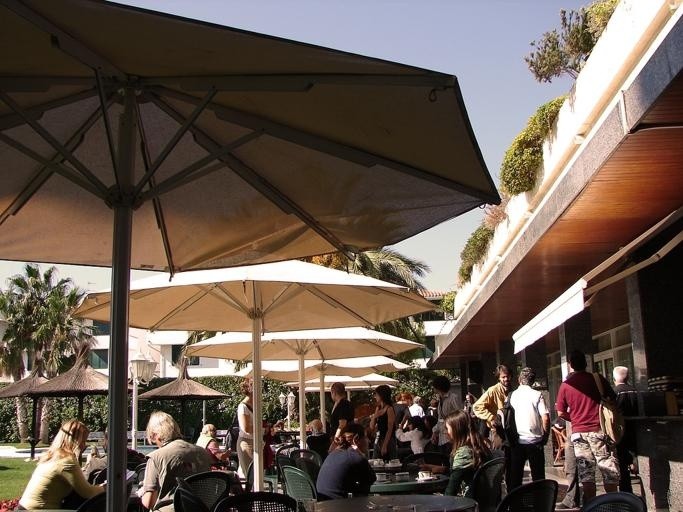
[71,426,647,511]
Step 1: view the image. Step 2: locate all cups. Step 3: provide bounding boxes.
[418,470,430,478]
[368,458,401,466]
[375,472,386,481]
[395,472,408,481]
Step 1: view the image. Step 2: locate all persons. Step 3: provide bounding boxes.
[235,373,253,488]
[194,424,243,502]
[304,351,638,507]
[140,411,217,512]
[14,419,106,512]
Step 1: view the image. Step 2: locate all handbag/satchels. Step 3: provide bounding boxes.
[263,428,274,470]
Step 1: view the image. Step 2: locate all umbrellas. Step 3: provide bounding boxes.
[283,373,396,392]
[181,326,426,458]
[0,1,502,511]
[70,260,444,492]
[0,357,49,458]
[232,355,412,433]
[28,338,142,420]
[137,357,231,433]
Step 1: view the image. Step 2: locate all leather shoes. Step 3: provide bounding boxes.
[555,503,565,509]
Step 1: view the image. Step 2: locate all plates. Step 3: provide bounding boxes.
[416,477,433,481]
[375,478,392,483]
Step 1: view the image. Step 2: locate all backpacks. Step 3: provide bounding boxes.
[593,371,624,444]
[492,391,518,450]
